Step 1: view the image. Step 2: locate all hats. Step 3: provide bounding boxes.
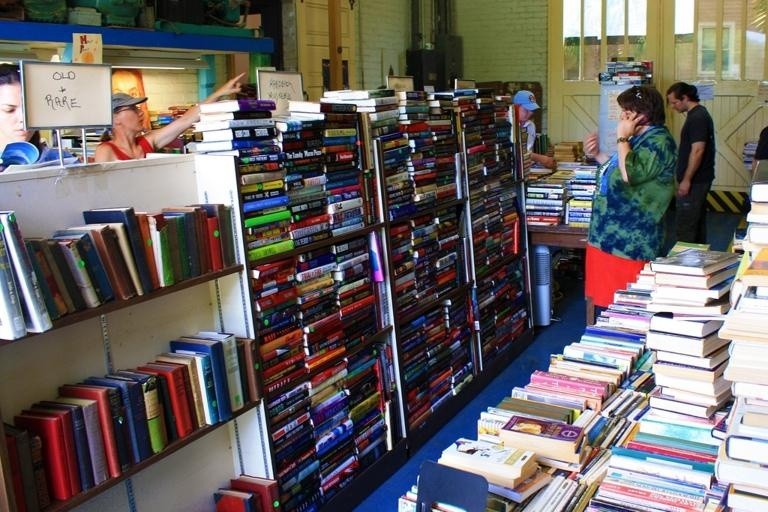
[512,89,541,112]
[112,92,148,110]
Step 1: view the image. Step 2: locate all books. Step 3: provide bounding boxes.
[526,140,599,229]
[191,92,532,510]
[0,333,263,510]
[397,182,768,511]
[742,142,758,171]
[598,57,653,84]
[59,103,204,154]
[212,474,280,512]
[2,206,232,341]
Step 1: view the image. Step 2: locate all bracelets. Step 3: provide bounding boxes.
[615,137,629,144]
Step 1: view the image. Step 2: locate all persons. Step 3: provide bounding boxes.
[94,72,247,161]
[514,90,555,168]
[665,81,715,245]
[0,63,82,172]
[580,86,680,309]
[752,126,768,177]
[111,69,142,111]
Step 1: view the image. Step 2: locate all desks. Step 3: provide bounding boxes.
[354,290,609,512]
[527,224,596,326]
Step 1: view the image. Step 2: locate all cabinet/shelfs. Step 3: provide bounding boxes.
[0,79,533,512]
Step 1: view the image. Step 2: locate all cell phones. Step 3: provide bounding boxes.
[634,113,650,126]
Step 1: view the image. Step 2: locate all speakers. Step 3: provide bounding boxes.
[407,48,446,92]
[437,34,463,89]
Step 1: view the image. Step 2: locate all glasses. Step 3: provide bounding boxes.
[631,86,644,106]
